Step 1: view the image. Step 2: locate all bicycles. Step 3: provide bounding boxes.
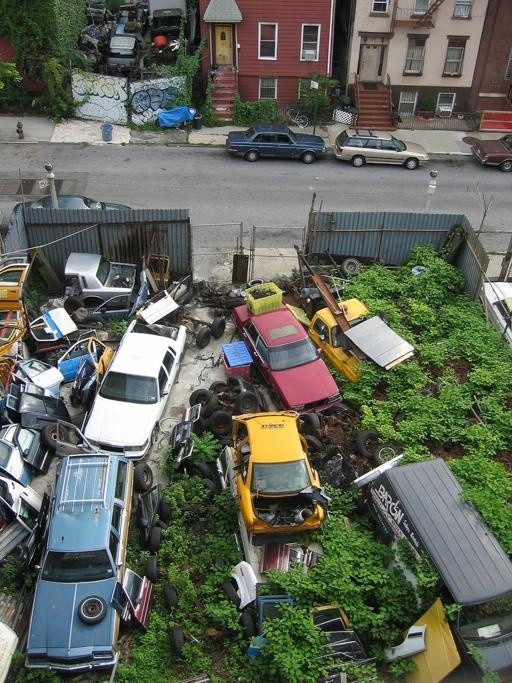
[282,106,308,128]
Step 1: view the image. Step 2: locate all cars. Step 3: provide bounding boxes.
[232,410,326,535]
[13,196,133,211]
[226,125,326,164]
[231,305,343,414]
[334,129,429,170]
[471,134,512,172]
[108,23,140,66]
[24,455,135,672]
[82,320,186,462]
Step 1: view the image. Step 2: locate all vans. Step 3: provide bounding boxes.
[366,458,511,683]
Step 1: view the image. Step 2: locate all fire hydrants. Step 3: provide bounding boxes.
[16,120,24,139]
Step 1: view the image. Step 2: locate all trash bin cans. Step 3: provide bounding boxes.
[100,124,113,140]
